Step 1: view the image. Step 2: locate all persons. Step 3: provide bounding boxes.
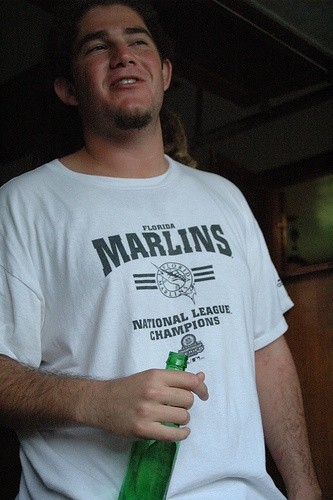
[1,1,323,499]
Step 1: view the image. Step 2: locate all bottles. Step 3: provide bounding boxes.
[117,351,189,500]
